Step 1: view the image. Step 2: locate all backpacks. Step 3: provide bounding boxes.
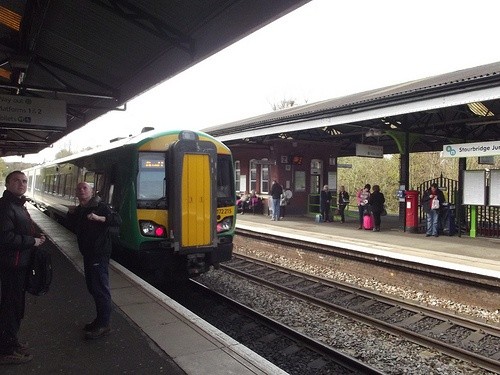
[109,208,122,235]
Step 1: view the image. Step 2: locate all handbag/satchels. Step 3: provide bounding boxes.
[343,198,350,203]
[333,215,342,222]
[25,245,53,297]
[381,209,387,216]
[315,213,322,222]
[432,197,439,210]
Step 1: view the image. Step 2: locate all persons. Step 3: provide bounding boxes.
[235,180,386,232]
[0,170,47,365]
[67,181,123,340]
[422,183,445,237]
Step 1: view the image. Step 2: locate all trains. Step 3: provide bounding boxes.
[20,126,238,286]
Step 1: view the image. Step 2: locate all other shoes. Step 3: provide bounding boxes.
[276,219,279,221]
[280,217,283,219]
[0,351,34,365]
[85,318,101,330]
[270,218,275,220]
[85,326,112,339]
[426,234,431,236]
[240,212,244,215]
[358,227,362,229]
[14,342,29,351]
[373,226,376,231]
[377,226,380,231]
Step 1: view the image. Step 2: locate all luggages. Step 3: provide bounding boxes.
[363,214,373,229]
